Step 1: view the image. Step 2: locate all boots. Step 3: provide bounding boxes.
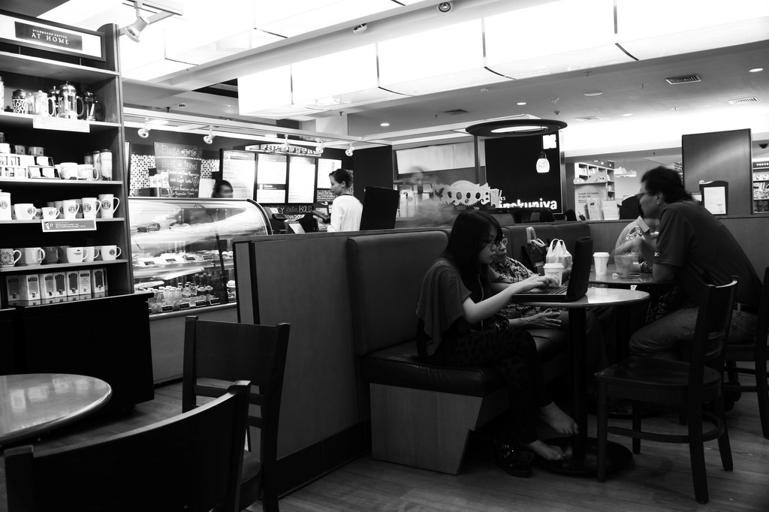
[504,440,533,478]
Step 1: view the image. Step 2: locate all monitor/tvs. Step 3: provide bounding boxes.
[287,221,305,234]
[361,187,400,231]
[485,131,563,214]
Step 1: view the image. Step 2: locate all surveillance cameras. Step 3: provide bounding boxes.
[759,144,767,148]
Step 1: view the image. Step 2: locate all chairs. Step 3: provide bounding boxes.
[591,268,741,503]
[1,378,253,511]
[672,263,768,442]
[177,313,290,511]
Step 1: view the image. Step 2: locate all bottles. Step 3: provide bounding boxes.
[83,146,112,180]
[147,280,196,307]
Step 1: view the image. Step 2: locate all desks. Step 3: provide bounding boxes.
[0,372,114,452]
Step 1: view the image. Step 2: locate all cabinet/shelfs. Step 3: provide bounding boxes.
[0,49,135,313]
[565,162,615,201]
[126,196,274,387]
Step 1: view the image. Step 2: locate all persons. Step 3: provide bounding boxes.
[210,179,233,199]
[416,208,580,463]
[485,230,569,328]
[607,165,764,426]
[327,168,365,232]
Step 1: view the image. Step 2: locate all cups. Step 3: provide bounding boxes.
[543,262,564,288]
[1,243,123,268]
[57,162,100,180]
[12,192,122,220]
[592,252,610,275]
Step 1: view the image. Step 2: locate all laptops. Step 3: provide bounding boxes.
[511,236,593,303]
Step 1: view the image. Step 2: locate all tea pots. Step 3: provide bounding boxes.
[12,81,106,119]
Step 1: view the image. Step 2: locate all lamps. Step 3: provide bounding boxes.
[536,125,550,174]
[123,0,148,43]
[121,105,396,157]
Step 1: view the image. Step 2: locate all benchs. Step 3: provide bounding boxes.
[344,218,592,477]
